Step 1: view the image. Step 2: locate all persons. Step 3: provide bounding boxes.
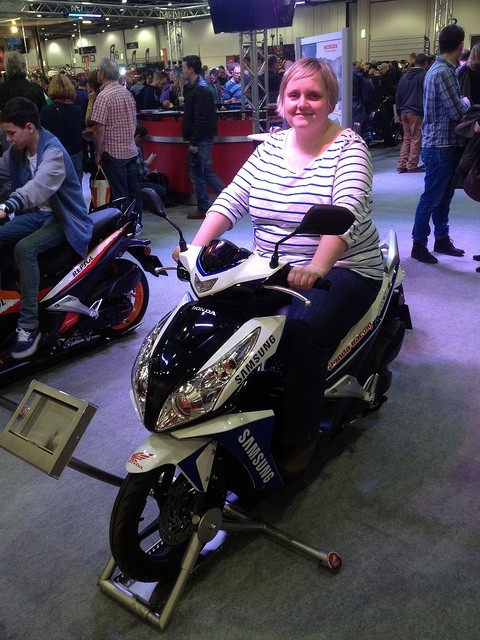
[142,71,170,109]
[40,74,85,188]
[217,65,228,85]
[0,96,95,359]
[223,63,264,110]
[203,65,208,79]
[454,104,480,272]
[455,49,470,76]
[427,53,436,65]
[146,75,153,86]
[206,68,220,112]
[91,58,143,227]
[410,24,471,264]
[30,75,37,83]
[352,59,407,146]
[75,76,89,109]
[182,55,226,219]
[171,57,384,480]
[65,74,71,80]
[395,54,428,172]
[258,54,283,145]
[127,62,139,77]
[226,63,233,80]
[134,125,167,210]
[170,73,186,111]
[409,52,417,64]
[60,70,66,76]
[459,43,480,105]
[37,76,49,95]
[71,80,77,88]
[0,51,48,112]
[25,68,43,76]
[164,68,171,77]
[130,75,145,97]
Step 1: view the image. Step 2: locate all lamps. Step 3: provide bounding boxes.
[11,20,18,34]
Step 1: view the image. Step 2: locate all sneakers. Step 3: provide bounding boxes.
[11,328,41,359]
[281,437,315,473]
[476,267,479,272]
[411,241,438,263]
[408,168,424,172]
[435,238,465,256]
[397,167,407,172]
[473,254,479,261]
[187,210,207,218]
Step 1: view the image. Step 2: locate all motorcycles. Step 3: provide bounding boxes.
[108,187,413,582]
[0,195,168,393]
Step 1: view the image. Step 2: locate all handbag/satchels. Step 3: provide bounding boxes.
[91,165,111,208]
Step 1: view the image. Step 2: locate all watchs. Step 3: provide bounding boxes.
[0,203,10,214]
